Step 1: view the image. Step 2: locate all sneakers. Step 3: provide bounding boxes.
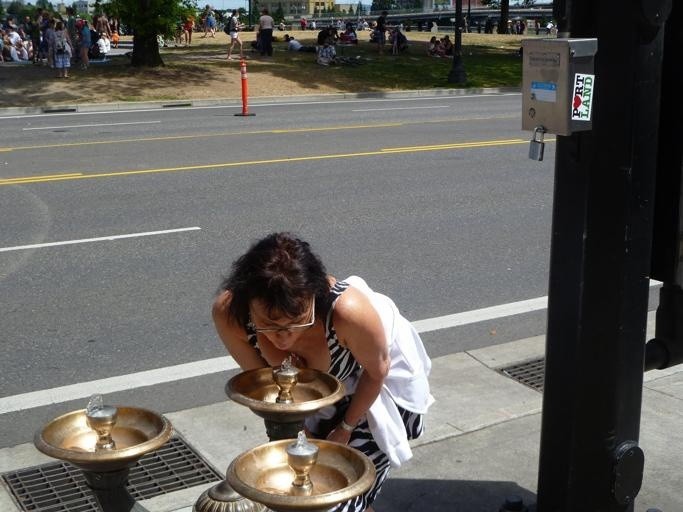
[56,75,69,78]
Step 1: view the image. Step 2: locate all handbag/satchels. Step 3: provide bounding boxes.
[224,23,230,34]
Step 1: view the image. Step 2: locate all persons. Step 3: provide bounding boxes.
[167,5,218,46]
[463,15,468,33]
[272,18,317,53]
[227,9,246,60]
[336,18,369,31]
[485,16,554,36]
[491,2,499,9]
[317,22,358,67]
[377,11,391,54]
[427,35,455,59]
[256,8,275,56]
[210,230,436,512]
[369,21,408,56]
[1,9,120,81]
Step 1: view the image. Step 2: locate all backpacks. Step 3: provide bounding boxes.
[54,33,65,54]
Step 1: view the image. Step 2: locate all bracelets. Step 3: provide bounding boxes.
[337,420,359,433]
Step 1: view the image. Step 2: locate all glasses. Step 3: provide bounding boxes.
[246,294,316,335]
